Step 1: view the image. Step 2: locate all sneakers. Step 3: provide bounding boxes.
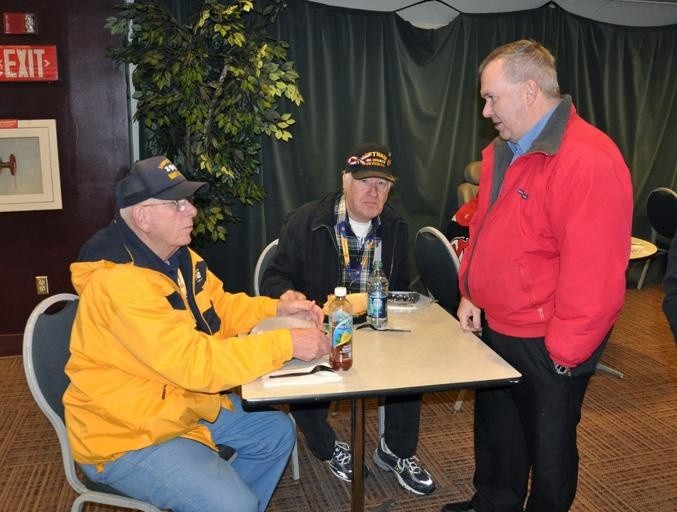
[373,435,436,496]
[325,440,370,483]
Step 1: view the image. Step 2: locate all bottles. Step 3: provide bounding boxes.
[363,235,390,328]
[328,286,354,372]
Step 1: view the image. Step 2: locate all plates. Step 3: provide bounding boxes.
[319,292,366,319]
[381,290,433,312]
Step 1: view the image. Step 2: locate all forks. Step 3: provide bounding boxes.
[355,324,411,332]
[268,364,333,379]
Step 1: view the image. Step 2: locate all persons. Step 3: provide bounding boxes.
[261,143,436,496]
[441,38,633,511]
[59,157,328,511]
[661,232,676,341]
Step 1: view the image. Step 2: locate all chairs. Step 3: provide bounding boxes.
[21,292,239,511]
[457,160,484,211]
[415,227,482,411]
[636,187,677,291]
[254,239,389,486]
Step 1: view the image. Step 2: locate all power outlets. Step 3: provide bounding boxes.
[35,276,49,295]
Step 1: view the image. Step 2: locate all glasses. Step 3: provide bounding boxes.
[143,199,186,214]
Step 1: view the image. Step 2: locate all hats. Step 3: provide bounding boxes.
[115,155,210,210]
[344,143,399,183]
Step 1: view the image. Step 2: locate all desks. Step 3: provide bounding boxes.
[596,236,658,378]
[241,290,522,512]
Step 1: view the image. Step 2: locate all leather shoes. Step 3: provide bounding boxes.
[441,500,476,512]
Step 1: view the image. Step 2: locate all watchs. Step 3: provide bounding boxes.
[555,364,569,374]
